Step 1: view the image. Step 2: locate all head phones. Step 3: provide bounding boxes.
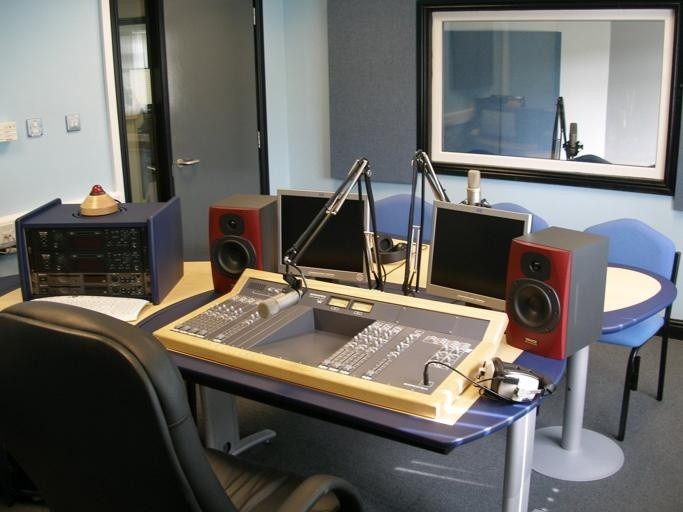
[479,357,556,404]
[371,232,406,264]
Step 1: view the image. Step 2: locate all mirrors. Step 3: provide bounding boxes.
[414,0,683,198]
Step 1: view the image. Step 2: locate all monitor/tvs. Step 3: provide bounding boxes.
[475,97,526,142]
[277,187,371,284]
[425,200,533,312]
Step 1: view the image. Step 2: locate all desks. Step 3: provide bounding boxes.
[369,230,677,482]
[0,260,568,512]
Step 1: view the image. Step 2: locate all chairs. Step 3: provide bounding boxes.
[0,300,368,511]
[475,202,550,239]
[367,192,435,245]
[584,216,681,442]
[461,98,529,154]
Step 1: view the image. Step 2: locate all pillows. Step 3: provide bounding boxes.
[480,109,516,142]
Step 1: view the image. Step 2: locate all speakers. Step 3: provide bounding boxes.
[209,193,278,295]
[505,226,608,361]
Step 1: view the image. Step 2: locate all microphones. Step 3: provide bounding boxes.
[568,123,577,155]
[421,360,510,403]
[467,170,481,205]
[257,290,304,319]
